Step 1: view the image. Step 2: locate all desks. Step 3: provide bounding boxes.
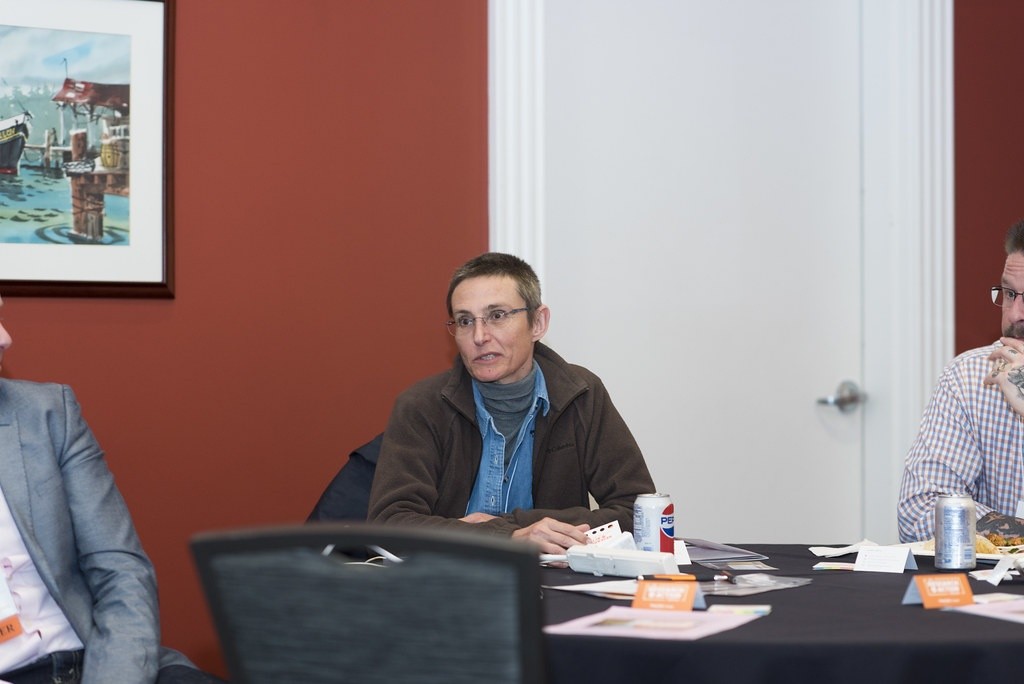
[544,544,1024,684]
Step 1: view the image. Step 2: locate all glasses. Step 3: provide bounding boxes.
[991,285,1024,308]
[446,307,528,336]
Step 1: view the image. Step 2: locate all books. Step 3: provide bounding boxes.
[679,537,770,562]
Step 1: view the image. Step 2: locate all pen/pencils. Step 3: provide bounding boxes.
[636,574,729,581]
[720,570,737,583]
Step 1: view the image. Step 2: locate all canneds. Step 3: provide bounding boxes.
[934,491,978,569]
[634,493,675,557]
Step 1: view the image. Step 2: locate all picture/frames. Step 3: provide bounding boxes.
[1,0,177,301]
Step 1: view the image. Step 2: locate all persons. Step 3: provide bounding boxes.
[896,220,1024,543]
[367,252,656,569]
[0,296,233,684]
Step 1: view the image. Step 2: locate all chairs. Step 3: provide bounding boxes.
[187,523,545,684]
